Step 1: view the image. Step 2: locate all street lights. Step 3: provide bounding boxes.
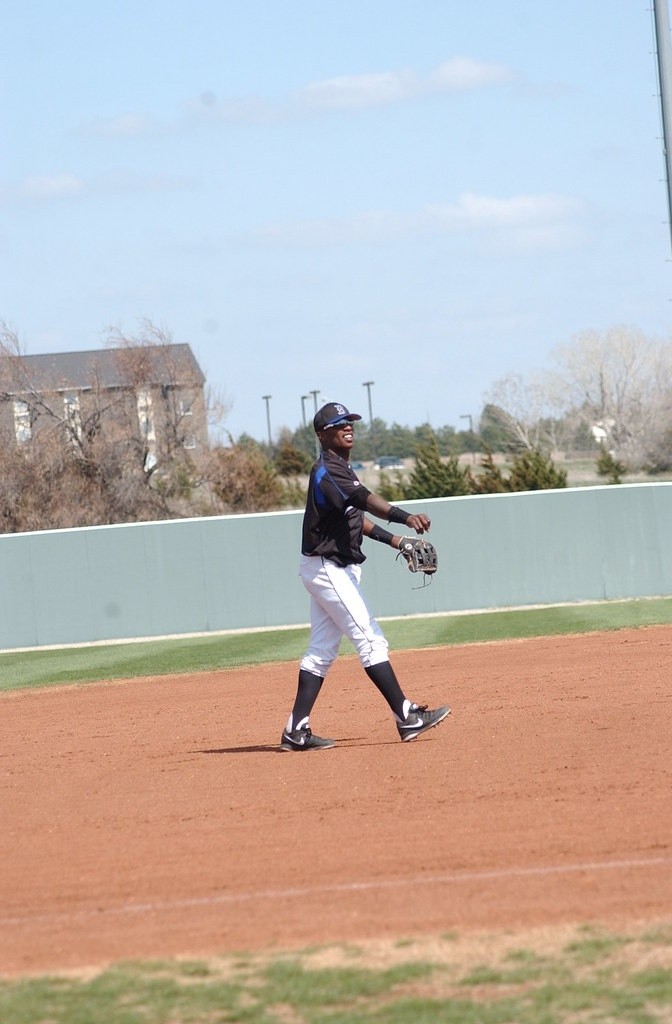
[262,395,272,448]
[363,381,375,424]
[459,414,473,433]
[301,396,311,430]
[310,390,320,413]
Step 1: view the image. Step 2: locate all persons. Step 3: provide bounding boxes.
[279,402,449,753]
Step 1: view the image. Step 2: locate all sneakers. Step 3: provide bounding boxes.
[396,701,451,742]
[280,727,335,752]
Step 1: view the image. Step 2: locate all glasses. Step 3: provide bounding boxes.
[318,419,354,437]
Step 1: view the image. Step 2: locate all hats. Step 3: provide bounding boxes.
[313,402,363,432]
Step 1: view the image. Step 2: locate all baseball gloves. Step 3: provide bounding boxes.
[398,536,439,575]
